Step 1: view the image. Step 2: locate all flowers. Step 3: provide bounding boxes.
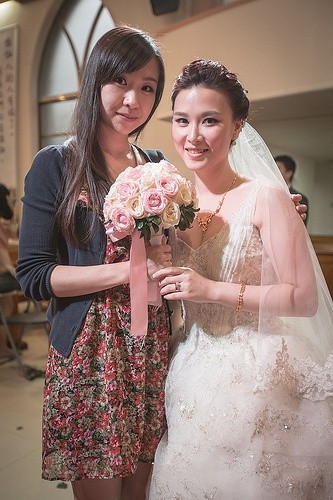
[104,159,200,337]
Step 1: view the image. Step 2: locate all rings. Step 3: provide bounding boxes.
[174,281,180,292]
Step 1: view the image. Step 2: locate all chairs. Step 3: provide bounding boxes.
[0,273,49,378]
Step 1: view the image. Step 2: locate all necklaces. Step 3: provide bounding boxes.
[193,173,237,237]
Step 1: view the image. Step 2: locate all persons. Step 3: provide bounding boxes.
[0,184,28,359]
[148,54,331,500]
[15,27,308,500]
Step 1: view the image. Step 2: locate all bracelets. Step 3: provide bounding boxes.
[235,283,245,311]
